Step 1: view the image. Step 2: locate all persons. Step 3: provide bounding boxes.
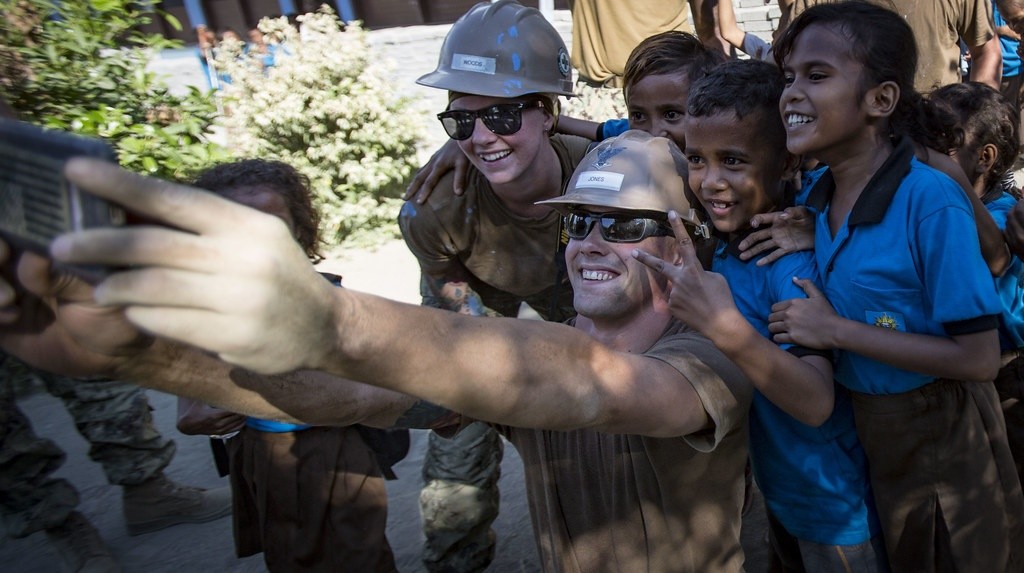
[174,159,397,572]
[397,2,600,573]
[631,61,876,572]
[1,354,231,573]
[2,128,750,573]
[767,2,1024,573]
[400,0,1024,573]
[200,27,287,92]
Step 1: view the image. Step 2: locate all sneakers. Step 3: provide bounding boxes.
[44,510,118,573]
[122,470,233,537]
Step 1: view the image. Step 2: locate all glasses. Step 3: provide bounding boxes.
[436,100,545,141]
[564,208,676,243]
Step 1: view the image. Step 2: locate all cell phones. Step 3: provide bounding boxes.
[0,116,127,287]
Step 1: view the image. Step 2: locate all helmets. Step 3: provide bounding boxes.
[533,129,714,240]
[415,0,577,98]
